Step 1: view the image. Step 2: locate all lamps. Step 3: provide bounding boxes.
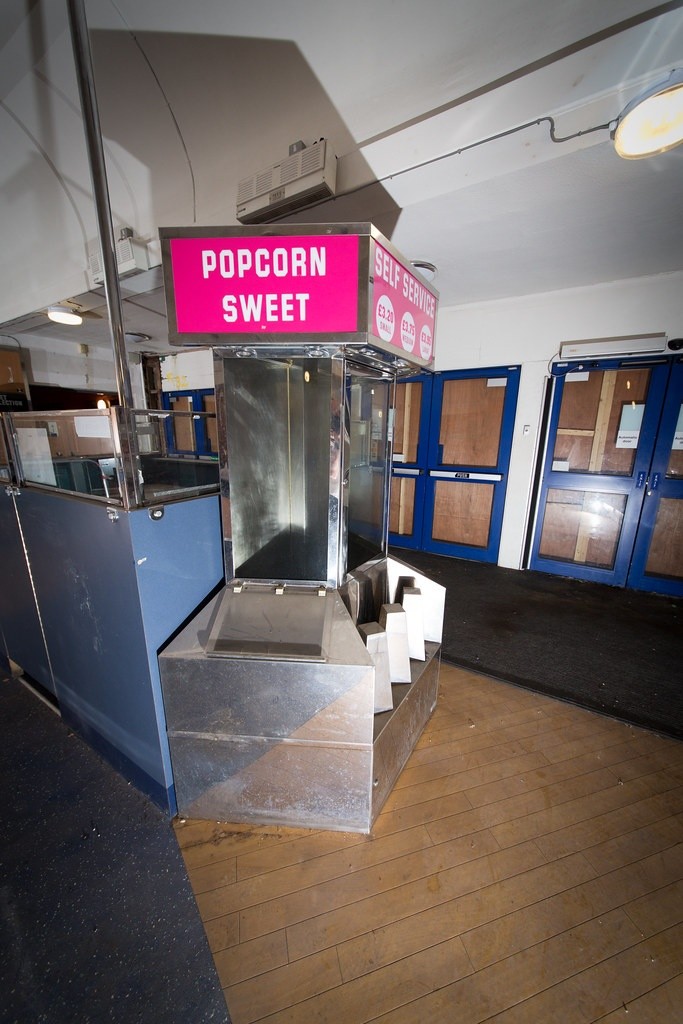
[605,68,683,161]
[47,306,83,325]
